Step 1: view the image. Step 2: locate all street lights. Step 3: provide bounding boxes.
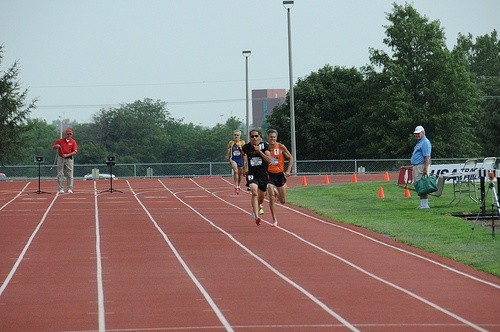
[283,1,294,175]
[242,50,251,144]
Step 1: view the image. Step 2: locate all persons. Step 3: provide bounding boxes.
[263,128,294,226]
[52,129,78,193]
[224,130,246,194]
[410,125,432,210]
[242,130,272,226]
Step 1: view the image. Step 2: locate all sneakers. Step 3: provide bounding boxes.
[260,208,264,214]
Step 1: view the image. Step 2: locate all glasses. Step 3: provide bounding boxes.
[67,133,71,135]
[234,134,240,137]
[251,135,258,138]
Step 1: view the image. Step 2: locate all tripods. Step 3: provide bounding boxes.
[100,164,123,194]
[30,163,52,195]
[466,179,500,244]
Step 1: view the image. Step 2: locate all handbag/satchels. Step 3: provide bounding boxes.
[413,173,438,195]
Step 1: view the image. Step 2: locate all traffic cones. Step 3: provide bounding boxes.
[351,174,357,183]
[301,176,308,187]
[378,186,385,198]
[384,172,390,181]
[404,188,411,198]
[324,174,330,184]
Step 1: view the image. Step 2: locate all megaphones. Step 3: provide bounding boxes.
[106,155,115,165]
[34,156,45,163]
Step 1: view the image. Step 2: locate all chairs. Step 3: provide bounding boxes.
[448,157,497,207]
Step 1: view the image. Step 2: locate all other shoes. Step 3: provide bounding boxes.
[256,217,261,225]
[416,205,428,209]
[67,190,72,193]
[235,188,240,195]
[59,190,65,194]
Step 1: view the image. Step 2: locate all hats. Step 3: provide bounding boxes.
[413,126,424,134]
[66,130,73,134]
